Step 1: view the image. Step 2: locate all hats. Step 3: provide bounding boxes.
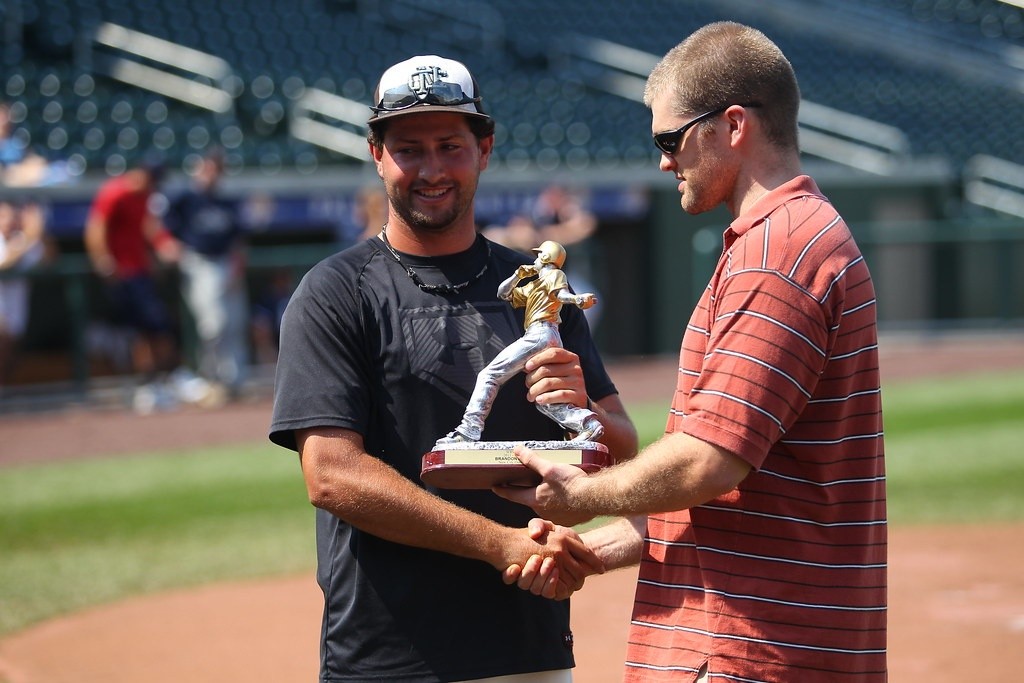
[367,55,492,127]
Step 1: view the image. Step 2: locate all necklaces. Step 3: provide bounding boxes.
[382,220,494,293]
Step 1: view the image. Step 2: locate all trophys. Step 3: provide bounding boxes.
[419,239,616,492]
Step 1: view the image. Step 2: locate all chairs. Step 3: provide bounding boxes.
[0,0,964,191]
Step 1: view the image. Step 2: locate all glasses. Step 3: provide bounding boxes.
[653,100,764,156]
[368,81,482,114]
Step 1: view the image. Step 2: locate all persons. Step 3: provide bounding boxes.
[0,101,597,421]
[492,20,887,683]
[268,54,638,682]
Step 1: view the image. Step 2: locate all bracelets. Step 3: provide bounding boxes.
[556,394,592,434]
